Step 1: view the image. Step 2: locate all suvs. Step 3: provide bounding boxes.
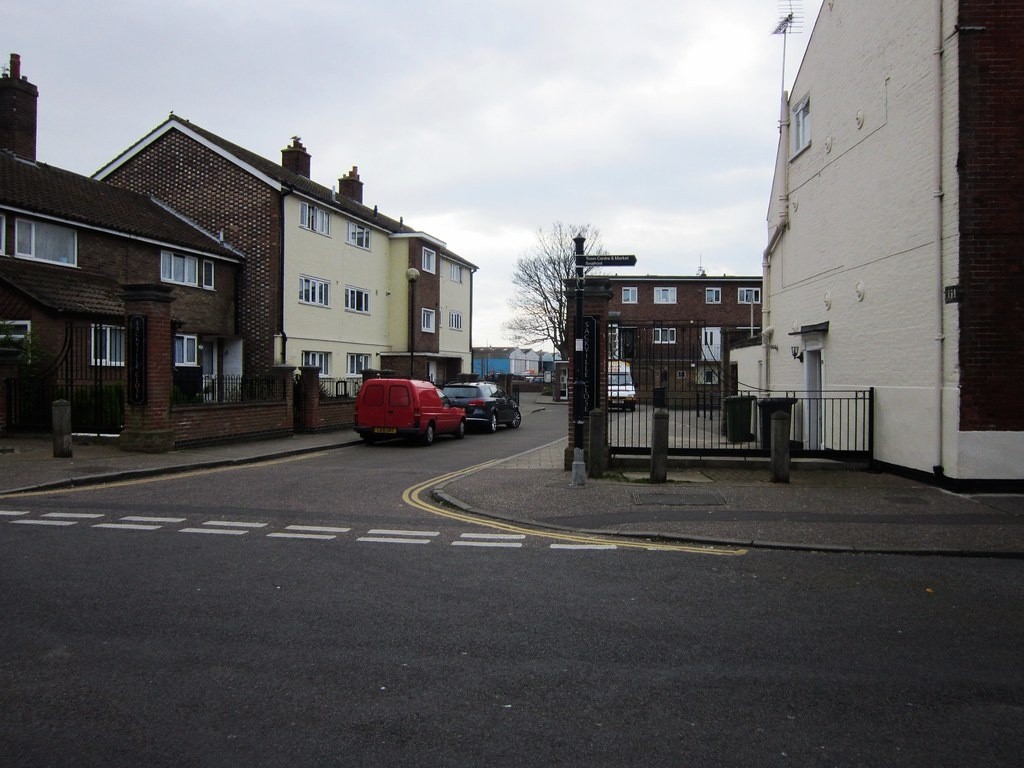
[442,380,522,434]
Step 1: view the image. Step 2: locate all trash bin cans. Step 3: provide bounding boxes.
[756,397,799,449]
[724,394,756,443]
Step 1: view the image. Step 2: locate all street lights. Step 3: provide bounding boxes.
[747,289,754,337]
[406,267,421,380]
[487,344,493,379]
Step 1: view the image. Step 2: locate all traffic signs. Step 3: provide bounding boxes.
[576,254,637,267]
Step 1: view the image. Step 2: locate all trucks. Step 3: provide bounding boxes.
[352,377,466,445]
[608,361,636,413]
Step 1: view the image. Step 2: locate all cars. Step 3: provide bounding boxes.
[531,377,545,383]
